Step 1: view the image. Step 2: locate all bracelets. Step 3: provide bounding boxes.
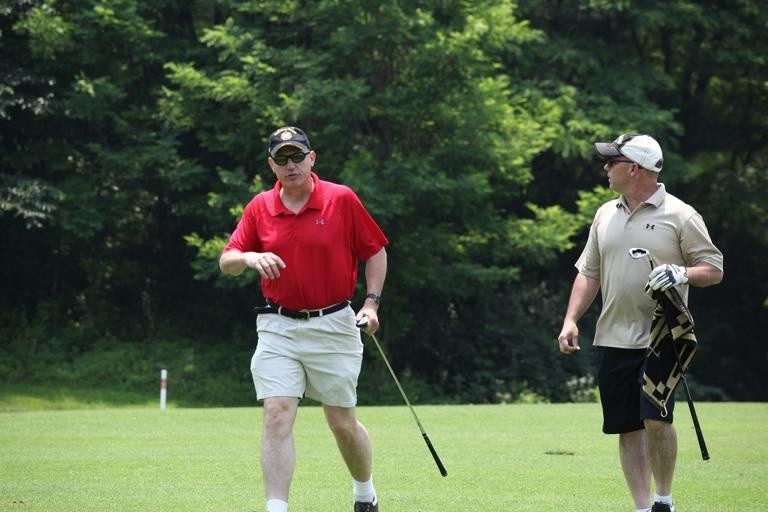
[363,293,382,304]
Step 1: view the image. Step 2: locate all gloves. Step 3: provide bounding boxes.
[648,263,688,292]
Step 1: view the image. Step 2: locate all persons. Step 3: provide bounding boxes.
[218,126,390,511]
[559,131,724,512]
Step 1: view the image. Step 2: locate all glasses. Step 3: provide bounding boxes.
[268,150,310,166]
[606,159,635,168]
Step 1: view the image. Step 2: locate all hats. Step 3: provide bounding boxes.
[594,134,664,174]
[267,126,311,154]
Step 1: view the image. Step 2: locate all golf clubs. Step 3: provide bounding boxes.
[628,247,710,461]
[356,316,448,477]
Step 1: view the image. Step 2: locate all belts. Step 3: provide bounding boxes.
[254,297,352,319]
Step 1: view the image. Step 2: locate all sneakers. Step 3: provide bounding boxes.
[354,495,379,512]
[651,500,675,512]
